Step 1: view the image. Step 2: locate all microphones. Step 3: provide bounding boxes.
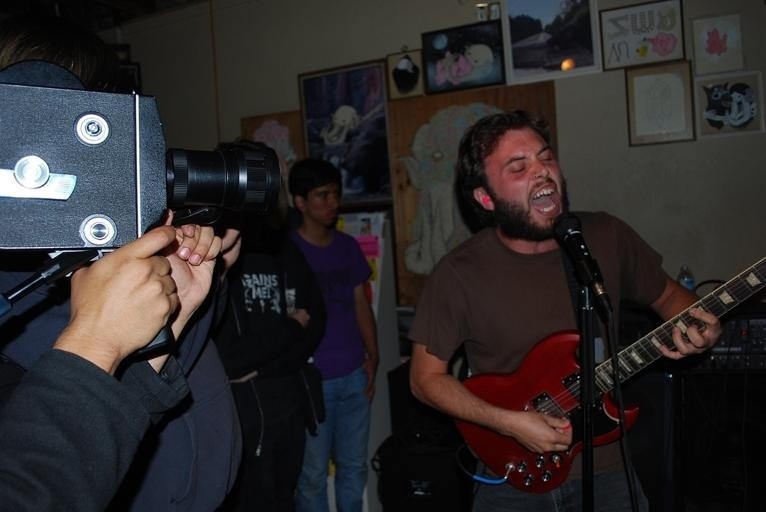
[551,212,612,322]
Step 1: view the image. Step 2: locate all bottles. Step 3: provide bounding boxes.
[674,263,695,295]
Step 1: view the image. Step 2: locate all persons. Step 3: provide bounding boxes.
[287,156,382,511]
[0,1,329,512]
[407,107,726,511]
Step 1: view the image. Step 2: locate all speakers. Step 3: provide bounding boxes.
[389,356,467,442]
[379,436,471,512]
[612,371,766,512]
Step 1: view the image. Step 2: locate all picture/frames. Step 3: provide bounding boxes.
[690,11,745,75]
[291,52,393,212]
[384,48,427,102]
[693,68,765,142]
[238,109,305,178]
[499,0,603,89]
[622,55,700,155]
[420,20,508,96]
[595,1,690,74]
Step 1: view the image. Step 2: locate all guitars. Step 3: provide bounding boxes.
[456,255,766,492]
